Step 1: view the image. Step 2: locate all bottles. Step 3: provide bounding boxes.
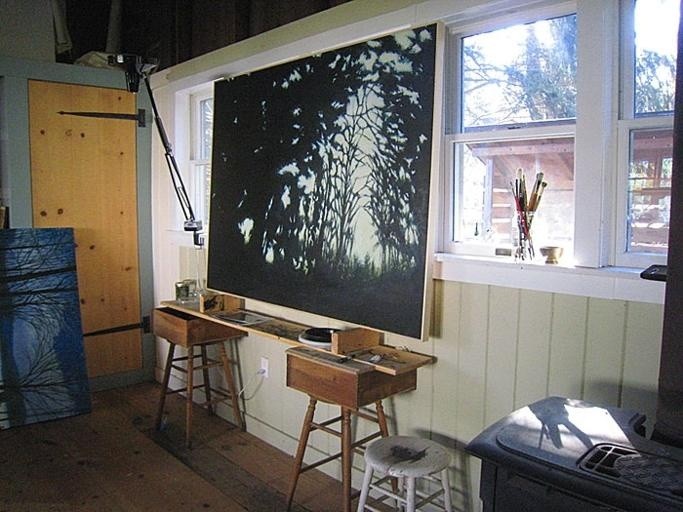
[183,279,195,297]
[174,282,188,306]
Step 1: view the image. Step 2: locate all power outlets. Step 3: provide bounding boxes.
[260,357,269,378]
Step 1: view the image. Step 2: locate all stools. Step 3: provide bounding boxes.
[287,387,401,511]
[356,433,457,512]
[154,340,246,447]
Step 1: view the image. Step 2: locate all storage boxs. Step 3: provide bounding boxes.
[154,308,248,346]
[284,347,420,408]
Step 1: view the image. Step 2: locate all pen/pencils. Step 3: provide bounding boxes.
[514,179,522,260]
[509,180,537,257]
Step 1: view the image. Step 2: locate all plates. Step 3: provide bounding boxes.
[304,328,341,341]
[297,332,330,347]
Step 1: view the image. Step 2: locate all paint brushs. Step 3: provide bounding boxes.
[528,182,548,230]
[523,174,530,213]
[516,172,544,260]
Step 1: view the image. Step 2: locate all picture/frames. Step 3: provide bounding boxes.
[203,18,444,342]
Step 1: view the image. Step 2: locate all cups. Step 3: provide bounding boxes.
[539,246,562,264]
